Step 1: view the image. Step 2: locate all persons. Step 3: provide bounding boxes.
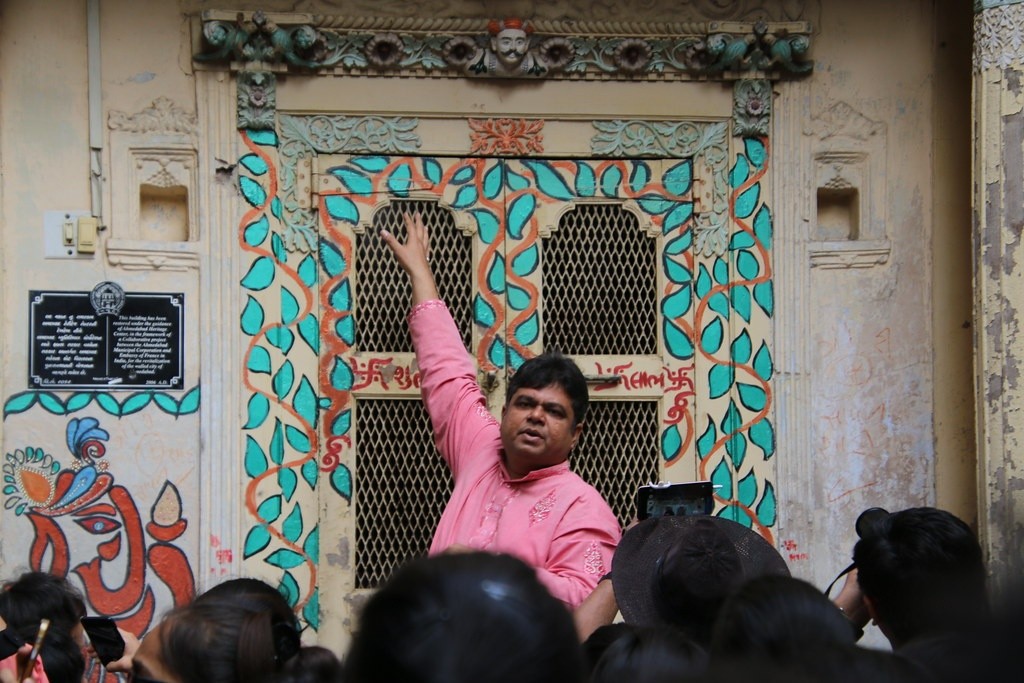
[483,19,533,77]
[380,212,623,612]
[0,506,1024,683]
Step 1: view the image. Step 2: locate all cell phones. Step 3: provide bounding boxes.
[80,616,126,667]
[20,619,50,683]
[637,481,715,521]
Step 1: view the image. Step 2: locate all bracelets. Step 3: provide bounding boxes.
[838,606,849,617]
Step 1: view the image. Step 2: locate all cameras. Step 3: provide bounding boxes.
[855,507,889,538]
